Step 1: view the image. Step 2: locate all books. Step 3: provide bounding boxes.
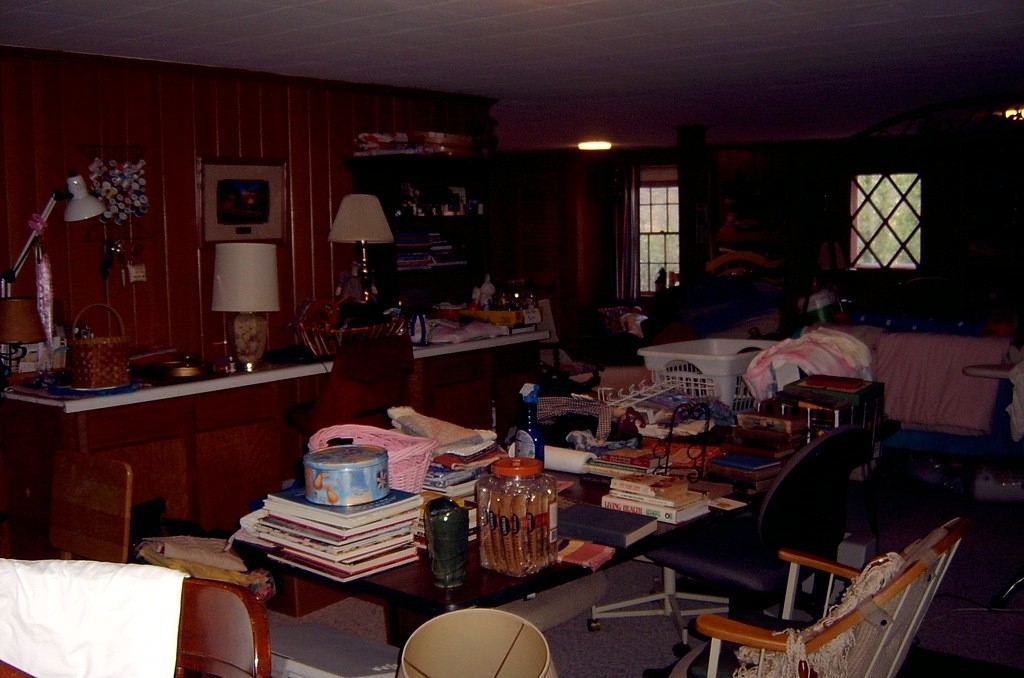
[413,453,487,549]
[557,502,658,549]
[396,232,468,270]
[705,451,783,496]
[719,373,884,457]
[601,471,711,524]
[257,484,425,582]
[585,447,670,478]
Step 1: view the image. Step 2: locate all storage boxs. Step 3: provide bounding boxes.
[637,338,778,413]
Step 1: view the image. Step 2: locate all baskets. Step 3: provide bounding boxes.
[307,424,440,495]
[637,337,782,414]
[70,304,130,388]
[293,318,404,357]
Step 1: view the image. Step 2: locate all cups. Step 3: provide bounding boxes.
[424,497,471,588]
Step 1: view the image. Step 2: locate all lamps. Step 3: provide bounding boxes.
[213,241,278,372]
[0,167,107,392]
[328,193,394,303]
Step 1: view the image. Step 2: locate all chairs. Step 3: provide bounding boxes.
[588,425,868,658]
[669,516,970,678]
[48,449,134,565]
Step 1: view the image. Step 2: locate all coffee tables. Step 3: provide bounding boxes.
[236,473,680,648]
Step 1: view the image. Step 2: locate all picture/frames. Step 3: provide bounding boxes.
[197,155,288,246]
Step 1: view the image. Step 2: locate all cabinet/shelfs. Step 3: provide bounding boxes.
[345,157,491,314]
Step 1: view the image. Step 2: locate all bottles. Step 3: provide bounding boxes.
[513,382,545,472]
